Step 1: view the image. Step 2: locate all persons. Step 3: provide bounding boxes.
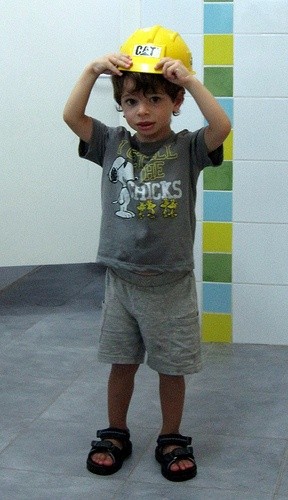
[63,23,232,482]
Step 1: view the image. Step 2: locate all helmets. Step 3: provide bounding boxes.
[114,26,192,80]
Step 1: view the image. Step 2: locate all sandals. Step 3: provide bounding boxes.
[155,433,197,482]
[86,427,132,476]
